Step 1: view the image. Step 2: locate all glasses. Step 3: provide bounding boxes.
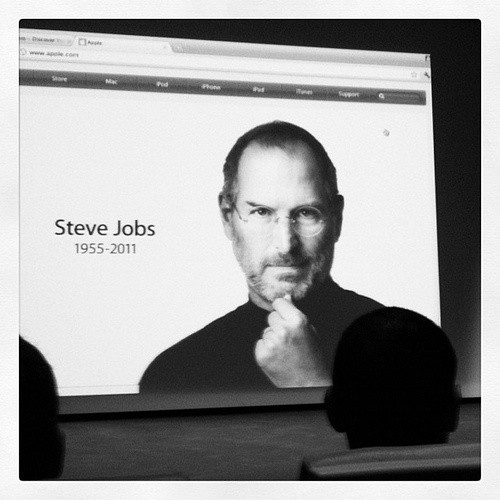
[230,198,340,236]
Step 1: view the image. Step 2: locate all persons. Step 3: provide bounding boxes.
[140,121,390,394]
[19,336,66,480]
[324,306,462,450]
